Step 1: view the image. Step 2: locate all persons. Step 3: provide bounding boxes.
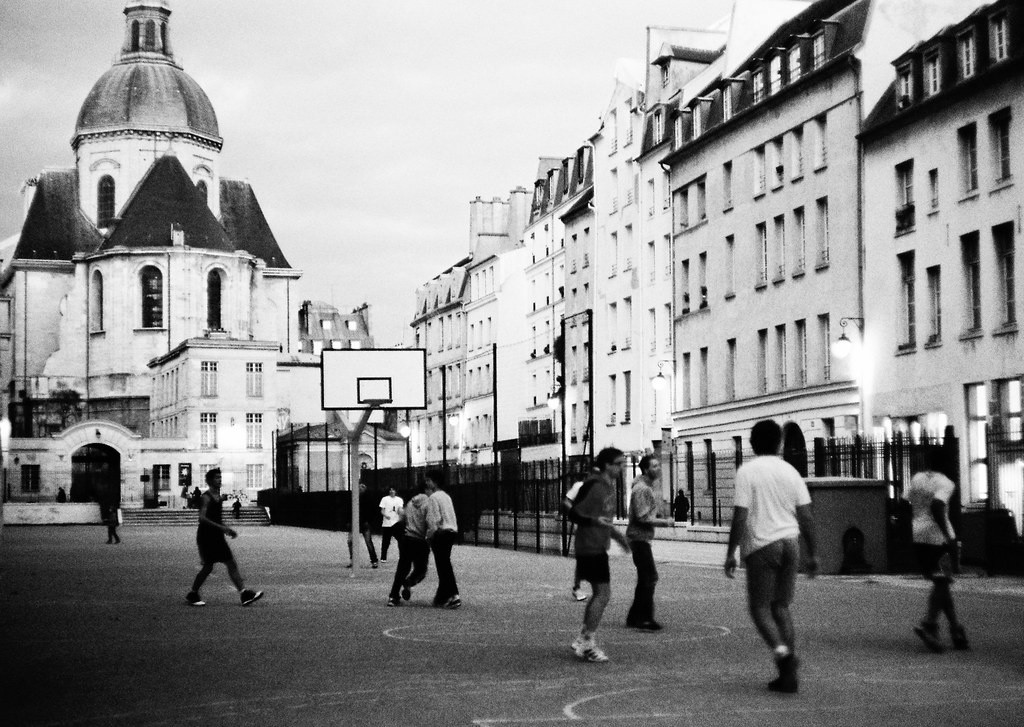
[56,487,66,503]
[186,468,264,606]
[896,446,970,652]
[563,447,630,663]
[626,455,665,631]
[388,486,435,607]
[379,485,404,563]
[675,489,690,522]
[423,469,462,609]
[345,482,379,569]
[725,420,821,693]
[94,464,121,544]
[182,481,242,519]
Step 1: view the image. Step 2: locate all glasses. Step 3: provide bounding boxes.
[613,461,627,467]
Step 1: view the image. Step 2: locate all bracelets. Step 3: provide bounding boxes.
[726,558,734,561]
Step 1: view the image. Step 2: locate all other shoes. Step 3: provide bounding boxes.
[106,541,112,544]
[345,563,352,568]
[241,590,263,606]
[767,653,802,693]
[432,599,450,608]
[949,624,968,650]
[372,562,378,568]
[114,540,121,544]
[913,615,944,653]
[445,599,461,609]
[402,578,411,601]
[184,593,205,606]
[387,597,403,606]
[381,560,386,563]
[626,618,663,630]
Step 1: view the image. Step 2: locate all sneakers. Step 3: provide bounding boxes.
[575,643,609,661]
[572,586,587,600]
[571,637,605,655]
